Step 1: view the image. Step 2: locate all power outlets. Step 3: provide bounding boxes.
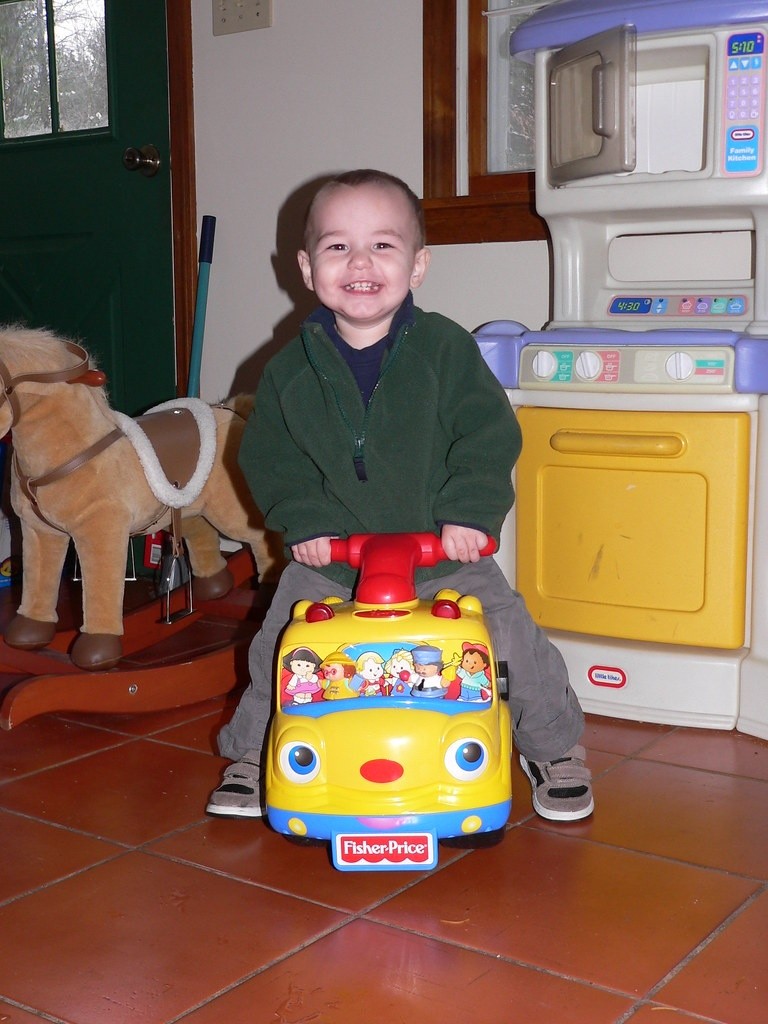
[212,0,273,36]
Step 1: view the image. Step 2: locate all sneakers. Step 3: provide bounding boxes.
[519,743,594,820]
[207,761,266,817]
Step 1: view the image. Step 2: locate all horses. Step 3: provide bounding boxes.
[0,324,285,671]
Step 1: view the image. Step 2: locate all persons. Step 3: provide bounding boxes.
[204,169,594,822]
